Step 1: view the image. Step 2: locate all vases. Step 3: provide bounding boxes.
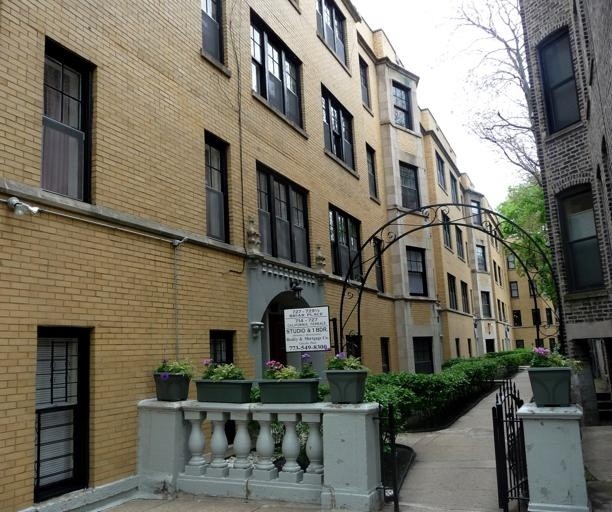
[527,366,571,408]
[153,370,369,404]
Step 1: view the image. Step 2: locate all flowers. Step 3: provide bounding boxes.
[152,348,365,381]
[529,344,583,373]
